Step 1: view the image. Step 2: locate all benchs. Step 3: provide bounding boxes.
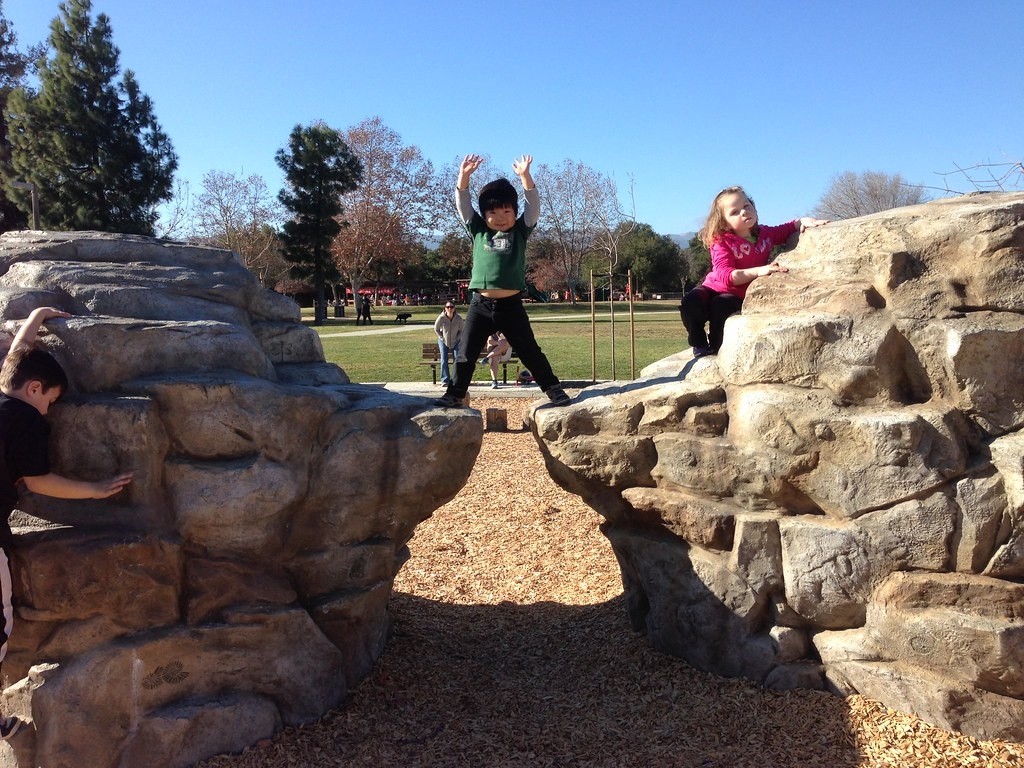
[420,344,518,384]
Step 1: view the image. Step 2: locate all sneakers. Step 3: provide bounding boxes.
[0,709,21,740]
[433,393,463,408]
[545,384,571,406]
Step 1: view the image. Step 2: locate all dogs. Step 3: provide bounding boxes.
[394,312,412,324]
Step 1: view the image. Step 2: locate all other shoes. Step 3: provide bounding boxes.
[480,356,489,366]
[692,343,716,358]
[491,380,498,389]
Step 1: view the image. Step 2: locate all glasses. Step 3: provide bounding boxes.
[445,306,455,309]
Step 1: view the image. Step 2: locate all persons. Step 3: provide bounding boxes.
[678,185,831,357]
[479,329,513,390]
[431,154,572,412]
[0,305,134,744]
[433,300,465,388]
[315,293,430,325]
[550,280,630,302]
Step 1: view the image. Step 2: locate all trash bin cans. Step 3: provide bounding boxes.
[334,305,345,317]
